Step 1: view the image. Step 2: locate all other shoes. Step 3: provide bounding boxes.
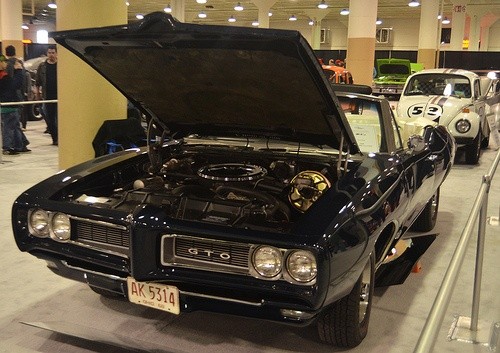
[19,147,31,153]
[3,148,19,155]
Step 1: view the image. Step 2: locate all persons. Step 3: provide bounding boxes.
[0,43,59,155]
[320,57,346,68]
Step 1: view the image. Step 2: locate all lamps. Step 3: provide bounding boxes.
[197,10,208,18]
[408,0,420,7]
[289,14,299,21]
[309,19,313,25]
[340,5,350,16]
[164,3,172,13]
[234,2,244,11]
[252,16,260,26]
[317,0,329,9]
[376,17,382,24]
[228,13,237,23]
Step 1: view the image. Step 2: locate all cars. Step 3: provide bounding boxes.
[396,67,500,165]
[371,57,424,98]
[10,10,456,349]
[21,54,48,121]
[319,64,365,116]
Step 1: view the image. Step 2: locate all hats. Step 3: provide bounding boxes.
[0,54,9,61]
